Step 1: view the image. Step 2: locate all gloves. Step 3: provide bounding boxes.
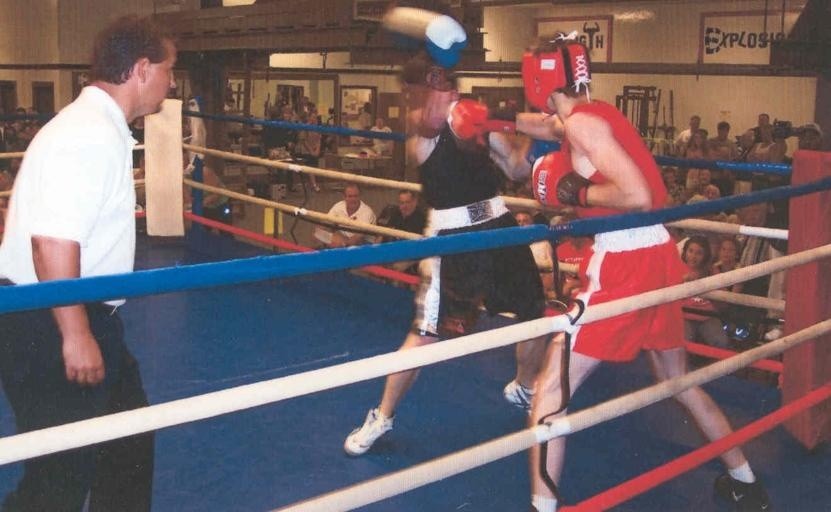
[523,140,592,208]
[447,98,517,141]
[424,14,466,69]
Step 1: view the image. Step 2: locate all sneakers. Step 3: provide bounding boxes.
[764,328,782,340]
[503,379,534,413]
[344,408,395,456]
[712,474,769,511]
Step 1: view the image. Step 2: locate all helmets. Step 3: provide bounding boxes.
[522,41,589,116]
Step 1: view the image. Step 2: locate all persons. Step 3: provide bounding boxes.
[128,113,144,210]
[0,20,176,512]
[448,31,770,512]
[0,105,57,242]
[225,101,426,288]
[344,5,549,456]
[184,150,235,238]
[497,115,825,388]
